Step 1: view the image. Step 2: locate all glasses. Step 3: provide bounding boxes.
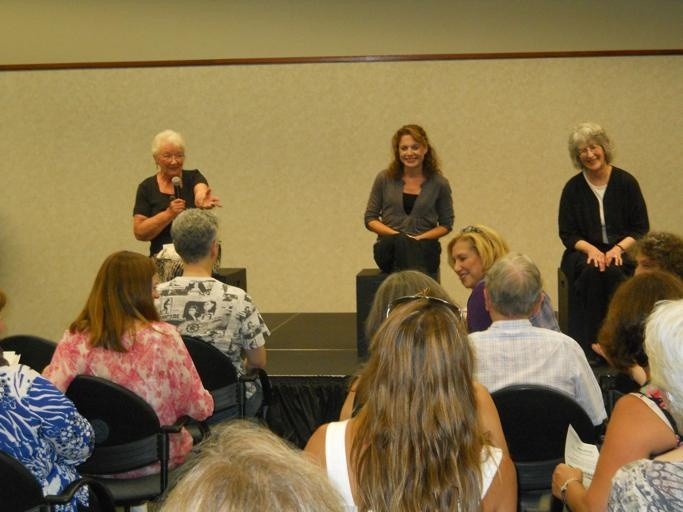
[578,144,601,156]
[381,296,461,327]
[157,152,185,161]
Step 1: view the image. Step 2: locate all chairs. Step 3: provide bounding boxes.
[488,384,602,512]
[1,336,276,511]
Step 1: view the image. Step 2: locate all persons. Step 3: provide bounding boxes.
[558,124,650,366]
[364,124,454,278]
[133,130,222,283]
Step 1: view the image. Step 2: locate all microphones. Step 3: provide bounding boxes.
[172,176,181,197]
